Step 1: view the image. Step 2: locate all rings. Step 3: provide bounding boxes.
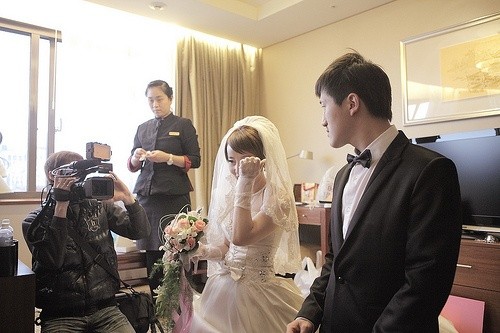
[63,183,67,185]
[67,185,69,187]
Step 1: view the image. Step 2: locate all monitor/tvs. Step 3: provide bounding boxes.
[408,128,500,240]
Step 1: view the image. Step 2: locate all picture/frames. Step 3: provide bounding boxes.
[400,11,500,127]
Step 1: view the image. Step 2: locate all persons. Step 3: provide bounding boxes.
[22,151,151,333]
[0,132,13,193]
[286,53,462,333]
[173,115,305,333]
[127,80,201,330]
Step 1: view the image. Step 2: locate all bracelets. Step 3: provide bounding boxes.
[167,154,173,165]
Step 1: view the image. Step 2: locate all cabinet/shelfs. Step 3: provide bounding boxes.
[450,239,500,333]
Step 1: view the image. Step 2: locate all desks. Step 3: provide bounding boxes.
[295,206,331,258]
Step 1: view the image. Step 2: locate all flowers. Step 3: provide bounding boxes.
[145,205,211,333]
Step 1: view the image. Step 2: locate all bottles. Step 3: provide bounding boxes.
[0,219,14,247]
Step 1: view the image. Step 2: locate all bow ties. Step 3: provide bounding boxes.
[347,149,372,169]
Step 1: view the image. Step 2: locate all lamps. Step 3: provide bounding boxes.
[286,151,314,161]
